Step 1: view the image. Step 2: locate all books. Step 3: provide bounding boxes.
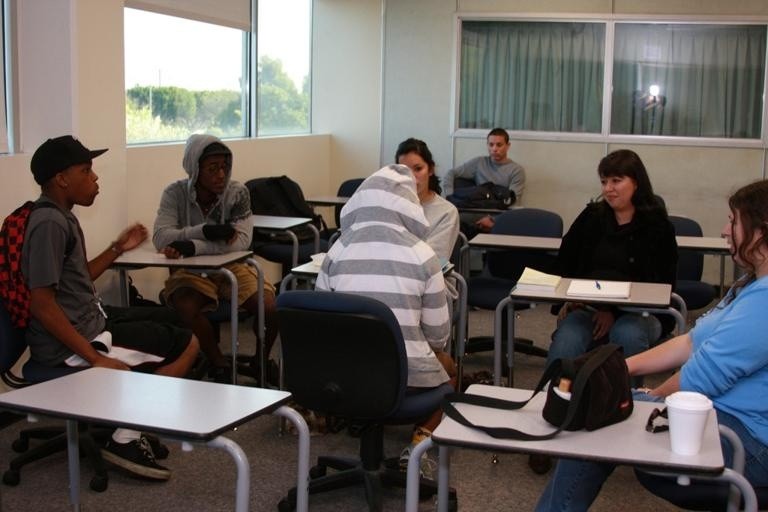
[515,266,562,293]
[566,276,633,302]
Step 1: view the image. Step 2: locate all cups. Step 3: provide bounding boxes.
[665,391,713,456]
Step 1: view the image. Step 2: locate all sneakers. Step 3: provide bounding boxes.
[529,453,552,473]
[398,444,435,480]
[250,357,279,388]
[99,433,170,479]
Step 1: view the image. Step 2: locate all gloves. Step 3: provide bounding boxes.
[203,224,235,242]
[167,240,195,257]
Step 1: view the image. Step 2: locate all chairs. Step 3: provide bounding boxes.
[317,178,369,242]
[453,177,477,190]
[241,176,341,266]
[464,207,564,359]
[271,290,457,512]
[667,215,715,309]
[250,213,321,272]
[303,210,306,211]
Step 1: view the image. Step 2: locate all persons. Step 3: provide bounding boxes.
[314,163,458,500]
[536,181,767,512]
[152,134,281,390]
[527,149,680,474]
[394,136,462,328]
[19,135,200,482]
[439,126,527,282]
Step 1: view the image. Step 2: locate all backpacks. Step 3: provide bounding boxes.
[0,199,78,330]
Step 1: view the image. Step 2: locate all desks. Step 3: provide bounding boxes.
[404,383,759,511]
[459,233,563,278]
[459,205,506,213]
[0,366,311,512]
[674,235,733,302]
[279,255,468,360]
[107,247,267,389]
[303,194,352,208]
[492,276,686,388]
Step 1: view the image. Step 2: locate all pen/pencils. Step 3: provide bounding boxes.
[595,278,601,291]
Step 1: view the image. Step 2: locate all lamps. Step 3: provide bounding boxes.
[643,85,666,113]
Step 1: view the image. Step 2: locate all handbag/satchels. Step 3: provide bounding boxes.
[442,342,635,442]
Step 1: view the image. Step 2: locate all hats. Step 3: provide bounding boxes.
[30,134,109,185]
